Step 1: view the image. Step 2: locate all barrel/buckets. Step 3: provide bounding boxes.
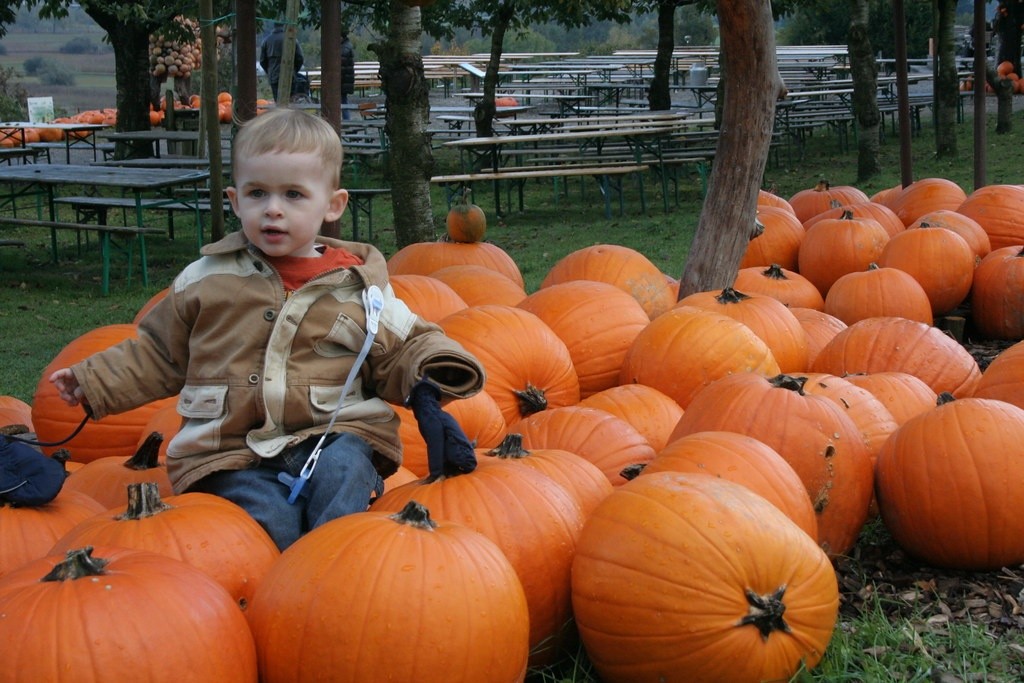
[27,97,55,124]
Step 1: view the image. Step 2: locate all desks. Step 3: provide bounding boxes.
[299,44,973,148]
[0,159,210,289]
[0,123,112,165]
[97,131,232,159]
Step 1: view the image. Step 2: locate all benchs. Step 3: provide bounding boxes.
[0,59,974,298]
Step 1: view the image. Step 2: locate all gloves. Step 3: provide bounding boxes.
[0,434,67,506]
[403,382,477,479]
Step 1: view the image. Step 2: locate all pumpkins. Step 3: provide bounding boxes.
[0,177,1024,683]
[494,97,518,117]
[958,62,1024,94]
[0,91,276,150]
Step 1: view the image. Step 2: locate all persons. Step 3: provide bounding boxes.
[49,108,485,554]
[260,23,305,103]
[340,25,355,134]
